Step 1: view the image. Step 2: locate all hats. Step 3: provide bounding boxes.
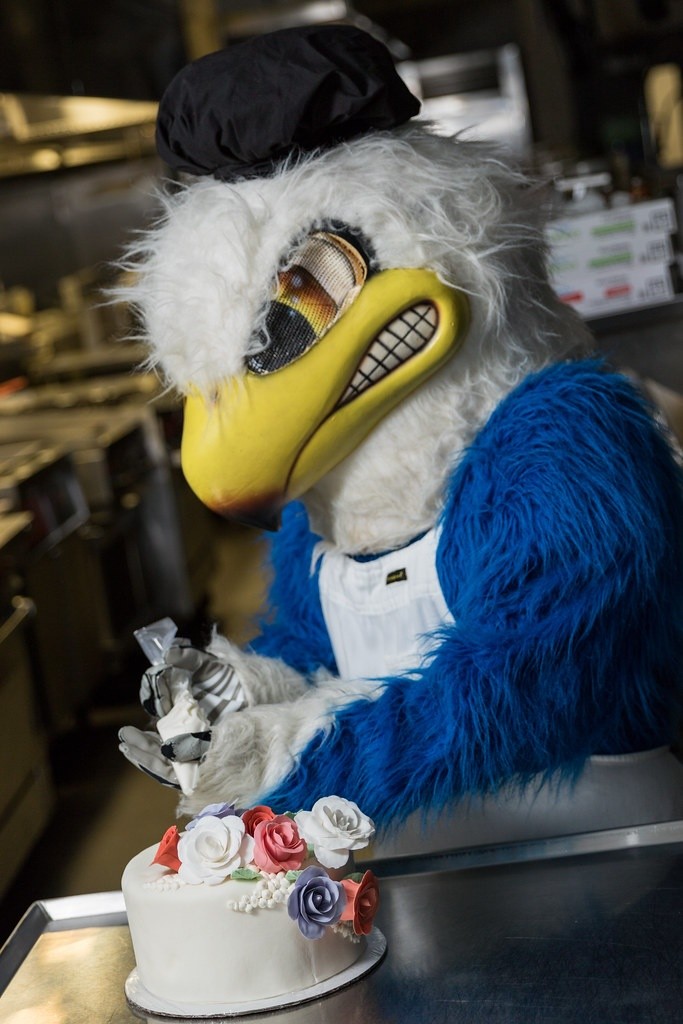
[156,24,422,184]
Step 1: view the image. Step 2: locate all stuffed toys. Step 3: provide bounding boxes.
[116,24,683,862]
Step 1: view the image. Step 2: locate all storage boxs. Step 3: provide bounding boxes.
[541,199,677,249]
[546,264,674,317]
[542,234,675,283]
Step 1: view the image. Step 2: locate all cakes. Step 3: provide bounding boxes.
[120,794,380,1004]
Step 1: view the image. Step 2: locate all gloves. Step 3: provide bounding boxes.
[139,645,247,727]
[120,727,213,799]
